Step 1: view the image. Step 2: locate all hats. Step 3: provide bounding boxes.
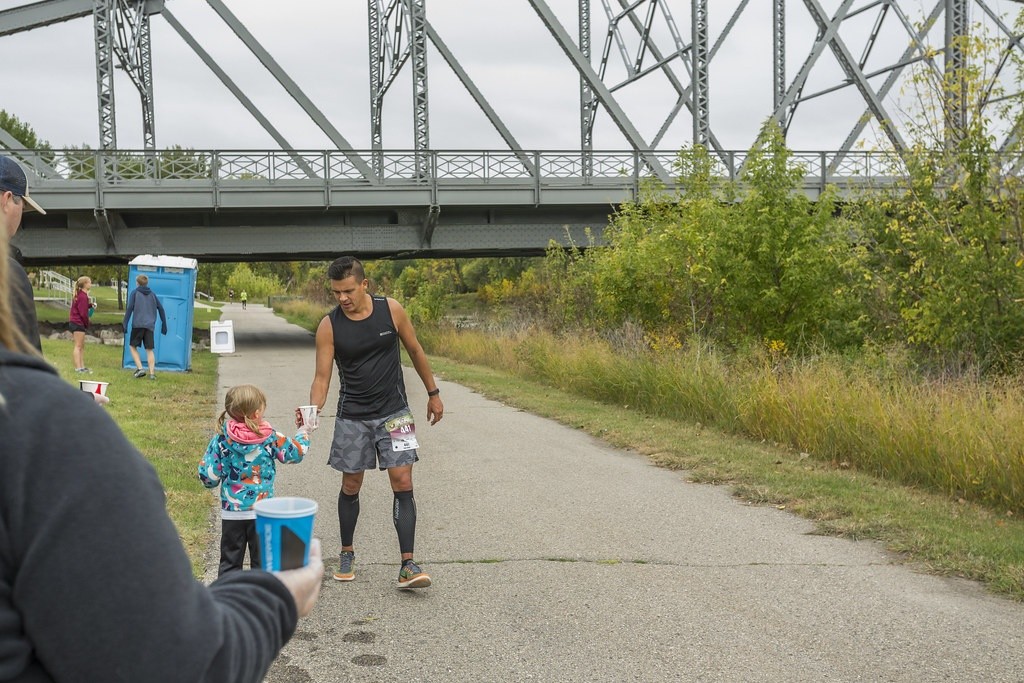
[0,155,46,215]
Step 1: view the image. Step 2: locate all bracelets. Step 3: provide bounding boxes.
[428,388,439,396]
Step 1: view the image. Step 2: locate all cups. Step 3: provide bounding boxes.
[252,497,319,573]
[78,380,109,406]
[298,405,318,427]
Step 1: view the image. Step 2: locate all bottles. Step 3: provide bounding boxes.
[88,305,96,318]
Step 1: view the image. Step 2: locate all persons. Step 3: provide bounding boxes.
[240,288,247,310]
[198,384,312,577]
[1,155,110,404]
[122,274,167,380]
[68,276,94,374]
[296,256,444,589]
[0,208,325,683]
[229,289,234,304]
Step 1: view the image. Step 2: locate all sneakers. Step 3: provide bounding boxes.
[150,374,157,379]
[395,560,432,590]
[333,551,356,581]
[133,368,146,378]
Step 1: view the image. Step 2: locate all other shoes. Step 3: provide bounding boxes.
[75,367,93,374]
